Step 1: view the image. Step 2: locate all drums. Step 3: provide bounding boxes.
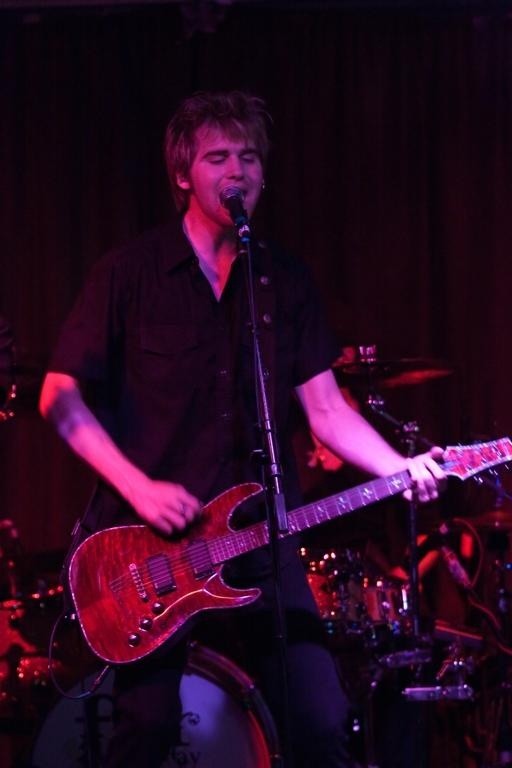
[301,547,362,622]
[338,575,413,637]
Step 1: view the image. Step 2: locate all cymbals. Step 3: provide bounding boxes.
[381,368,459,387]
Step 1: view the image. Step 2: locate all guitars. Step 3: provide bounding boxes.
[0,582,64,654]
[67,438,512,666]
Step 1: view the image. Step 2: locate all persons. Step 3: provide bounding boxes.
[299,378,413,543]
[37,93,447,767]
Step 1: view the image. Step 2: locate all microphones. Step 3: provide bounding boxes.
[219,185,250,242]
